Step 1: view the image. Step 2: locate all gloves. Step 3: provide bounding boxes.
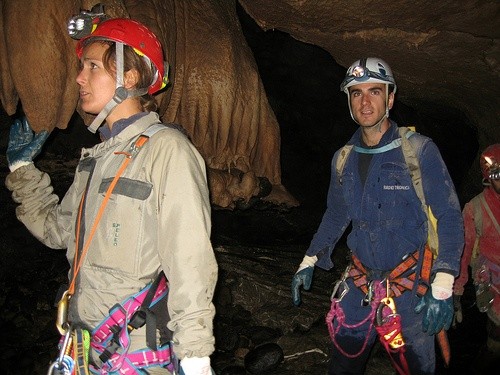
[177,357,214,375]
[449,295,463,326]
[291,254,318,304]
[414,272,455,335]
[6,119,48,172]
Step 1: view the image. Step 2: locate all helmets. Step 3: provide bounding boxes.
[481,144,500,179]
[341,57,395,91]
[76,19,165,95]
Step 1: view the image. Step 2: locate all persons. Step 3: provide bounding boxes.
[451,143,500,375]
[5,3,219,375]
[292,57,465,375]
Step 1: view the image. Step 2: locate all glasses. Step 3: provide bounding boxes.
[488,171,500,183]
